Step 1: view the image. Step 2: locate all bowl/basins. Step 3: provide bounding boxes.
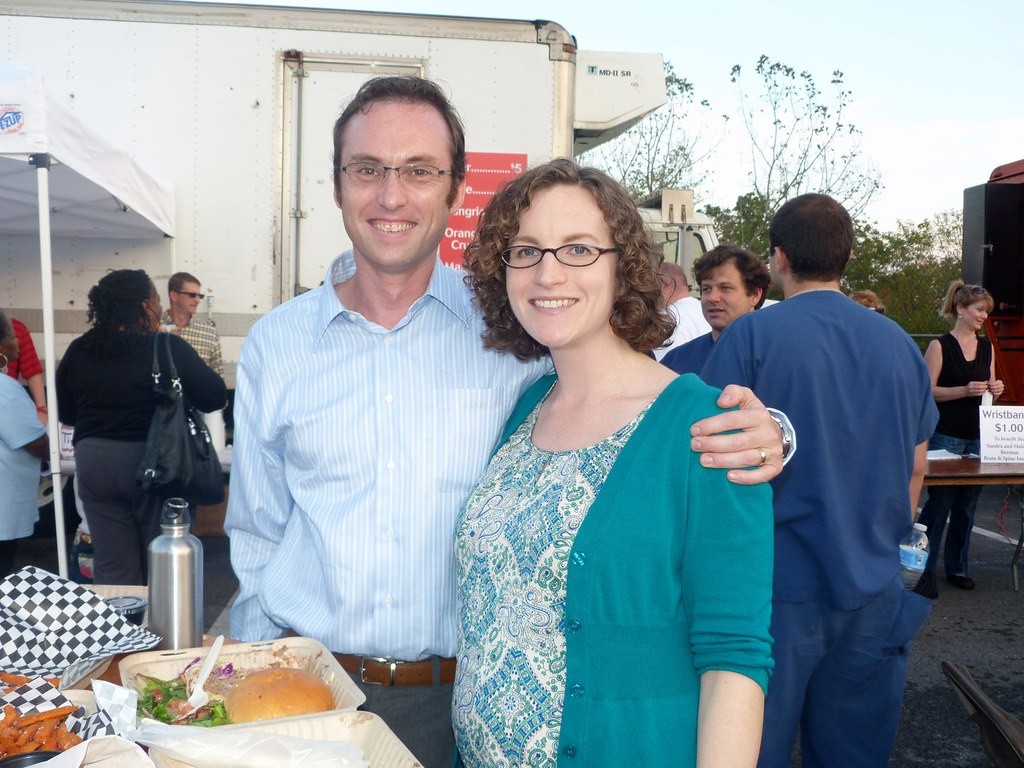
[101,594,148,625]
[0,750,66,768]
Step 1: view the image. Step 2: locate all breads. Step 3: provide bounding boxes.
[223,667,335,723]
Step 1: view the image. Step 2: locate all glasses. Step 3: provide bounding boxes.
[499,243,622,269]
[173,291,204,300]
[338,162,454,189]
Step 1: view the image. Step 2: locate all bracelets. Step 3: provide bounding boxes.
[37,405,47,413]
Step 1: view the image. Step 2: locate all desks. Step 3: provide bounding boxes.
[922,457,1024,592]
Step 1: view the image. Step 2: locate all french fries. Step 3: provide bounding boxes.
[0,670,82,761]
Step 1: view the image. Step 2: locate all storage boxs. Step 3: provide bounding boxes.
[60,584,424,768]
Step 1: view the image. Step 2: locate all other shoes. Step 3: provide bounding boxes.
[946,572,977,590]
[915,572,940,600]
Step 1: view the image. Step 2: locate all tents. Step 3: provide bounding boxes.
[0,82,175,576]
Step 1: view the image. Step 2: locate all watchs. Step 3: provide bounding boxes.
[769,411,792,460]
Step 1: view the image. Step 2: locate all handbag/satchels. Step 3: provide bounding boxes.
[135,332,224,506]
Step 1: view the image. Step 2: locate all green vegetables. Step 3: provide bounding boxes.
[132,672,229,727]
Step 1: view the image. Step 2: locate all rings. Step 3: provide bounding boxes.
[755,448,767,466]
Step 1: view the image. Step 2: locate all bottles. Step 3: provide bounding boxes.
[146,497,205,653]
[899,522,930,592]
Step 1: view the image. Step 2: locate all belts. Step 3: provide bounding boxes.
[284,627,457,686]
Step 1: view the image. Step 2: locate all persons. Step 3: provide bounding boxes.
[158,272,225,423]
[651,262,715,365]
[913,282,1004,599]
[659,245,773,377]
[848,291,887,315]
[224,79,797,768]
[0,307,51,587]
[698,193,940,768]
[453,158,775,768]
[55,271,228,627]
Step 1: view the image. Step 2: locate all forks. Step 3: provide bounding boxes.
[171,635,224,722]
